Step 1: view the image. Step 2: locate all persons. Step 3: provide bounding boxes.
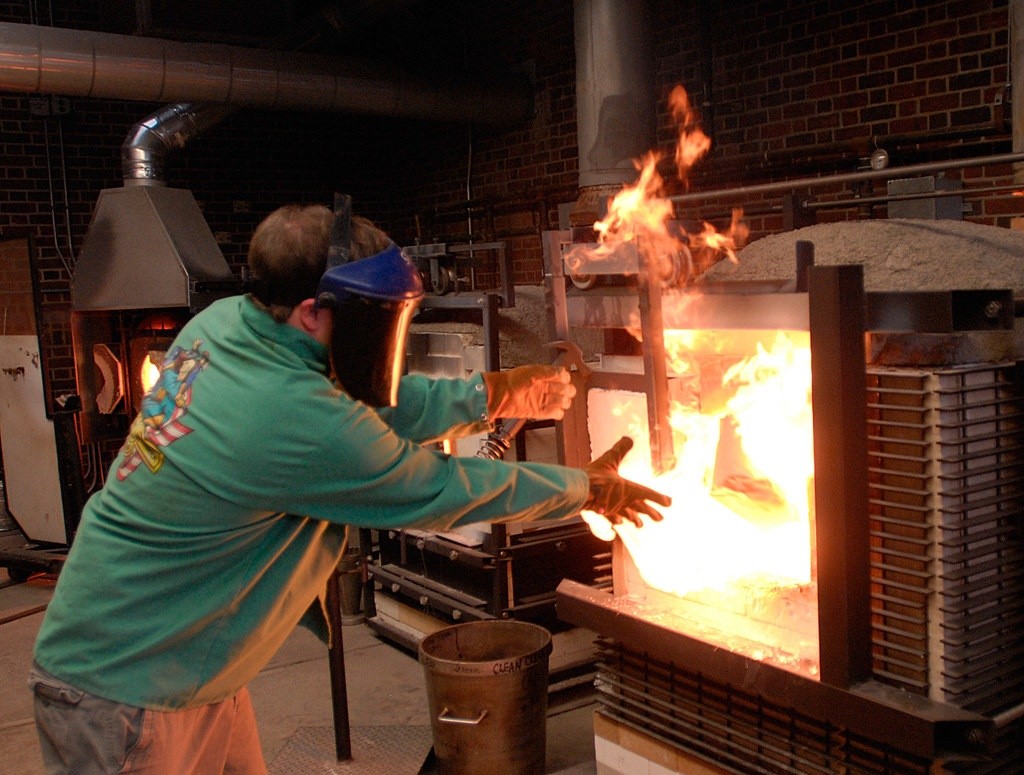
[28,206,673,775]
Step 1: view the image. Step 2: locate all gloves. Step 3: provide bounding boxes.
[584,436,671,528]
[476,364,577,421]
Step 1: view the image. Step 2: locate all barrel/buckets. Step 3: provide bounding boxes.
[418,621,554,775]
